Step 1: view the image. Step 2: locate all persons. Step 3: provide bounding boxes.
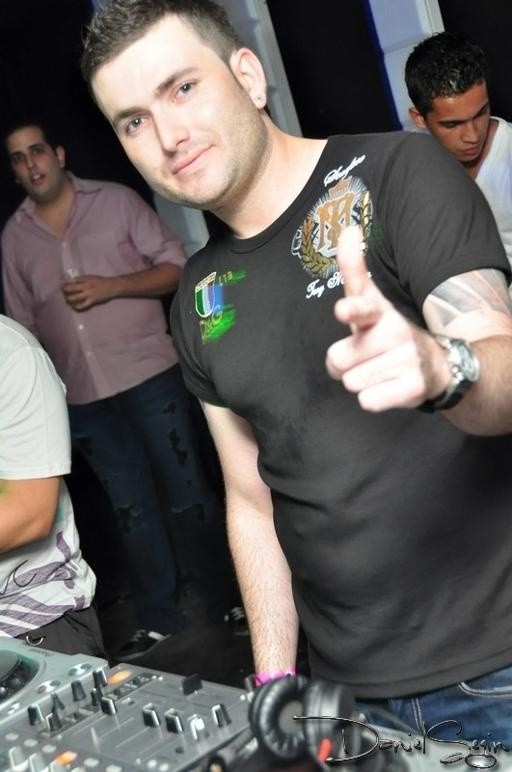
[0,304,108,665]
[402,33,512,302]
[66,0,510,754]
[0,108,258,668]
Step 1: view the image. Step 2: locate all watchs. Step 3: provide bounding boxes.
[417,332,480,414]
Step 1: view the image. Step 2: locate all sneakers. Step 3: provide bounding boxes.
[223,606,251,636]
[113,629,172,662]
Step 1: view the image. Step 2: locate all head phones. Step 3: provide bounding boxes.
[224,673,362,772]
